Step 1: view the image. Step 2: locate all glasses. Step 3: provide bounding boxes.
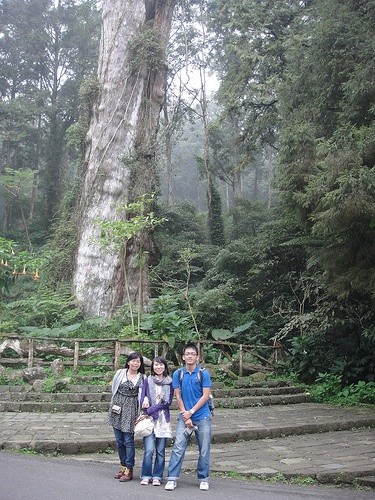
[184,353,197,357]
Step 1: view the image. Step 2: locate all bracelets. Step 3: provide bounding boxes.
[190,409,194,414]
[181,412,184,414]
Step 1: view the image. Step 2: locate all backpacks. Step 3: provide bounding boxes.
[179,369,213,414]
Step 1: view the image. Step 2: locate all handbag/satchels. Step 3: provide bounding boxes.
[134,414,154,437]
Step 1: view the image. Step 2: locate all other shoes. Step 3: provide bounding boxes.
[152,479,160,485]
[120,471,133,481]
[115,471,124,478]
[140,479,149,484]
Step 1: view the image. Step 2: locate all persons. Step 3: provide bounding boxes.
[108,353,148,482]
[140,356,174,485]
[164,342,212,490]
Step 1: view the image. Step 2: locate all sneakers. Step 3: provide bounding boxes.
[164,480,177,490]
[199,481,209,490]
